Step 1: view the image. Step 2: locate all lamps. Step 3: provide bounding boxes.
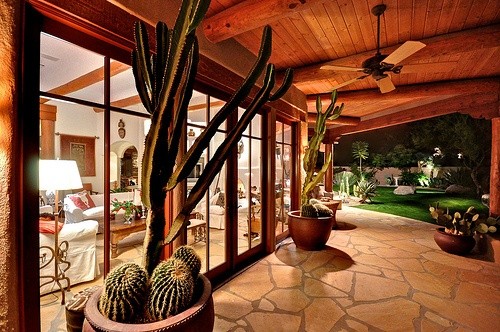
[39,159,83,305]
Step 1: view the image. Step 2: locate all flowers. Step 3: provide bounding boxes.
[110,198,140,219]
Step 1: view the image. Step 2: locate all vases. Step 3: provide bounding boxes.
[124,217,131,224]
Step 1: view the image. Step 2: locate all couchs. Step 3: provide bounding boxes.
[39,205,100,296]
[202,191,224,229]
[64,192,142,224]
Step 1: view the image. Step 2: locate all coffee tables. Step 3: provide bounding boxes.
[98,217,147,258]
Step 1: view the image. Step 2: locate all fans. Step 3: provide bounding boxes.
[320,4,426,94]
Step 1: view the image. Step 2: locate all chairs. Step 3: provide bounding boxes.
[243,204,281,237]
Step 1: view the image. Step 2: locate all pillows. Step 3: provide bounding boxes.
[67,190,96,211]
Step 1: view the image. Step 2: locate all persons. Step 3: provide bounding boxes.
[251,186,260,205]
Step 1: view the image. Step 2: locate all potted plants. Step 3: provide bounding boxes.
[82,0,295,332]
[429,202,496,254]
[287,90,345,251]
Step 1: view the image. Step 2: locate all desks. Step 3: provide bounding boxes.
[188,219,207,246]
